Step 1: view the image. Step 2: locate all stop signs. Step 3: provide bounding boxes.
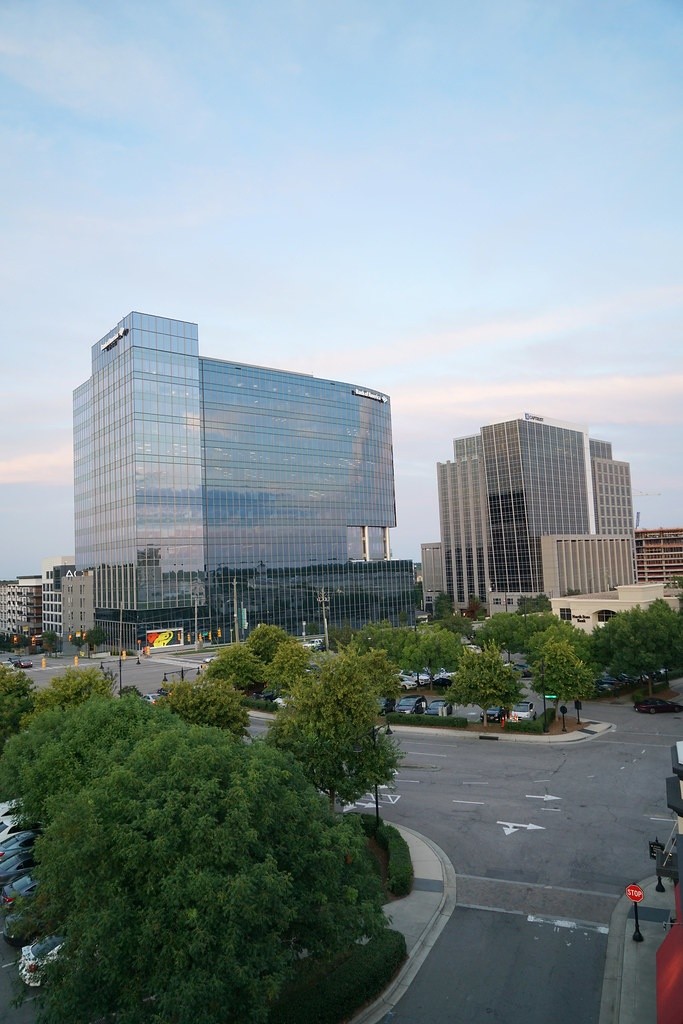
[626,883,644,903]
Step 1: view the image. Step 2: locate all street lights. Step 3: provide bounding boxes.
[162,667,200,683]
[354,721,394,835]
[99,657,141,693]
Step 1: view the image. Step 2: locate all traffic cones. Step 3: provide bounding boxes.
[500,713,520,728]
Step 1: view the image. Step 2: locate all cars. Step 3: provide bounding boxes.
[2,802,81,986]
[397,695,428,714]
[634,699,683,712]
[424,700,453,715]
[510,700,536,721]
[479,701,509,721]
[9,658,33,669]
[0,661,13,668]
[301,639,325,647]
[371,644,531,689]
[591,667,665,694]
[373,700,394,715]
[144,693,162,703]
